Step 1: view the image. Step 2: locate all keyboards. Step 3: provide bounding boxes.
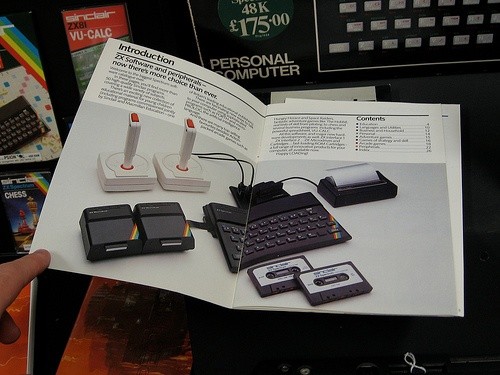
[203,191,353,267]
[1,95,48,157]
[314,0,499,72]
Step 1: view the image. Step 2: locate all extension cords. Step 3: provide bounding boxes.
[230,179,288,204]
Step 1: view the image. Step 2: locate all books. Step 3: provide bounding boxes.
[27,37,465,317]
